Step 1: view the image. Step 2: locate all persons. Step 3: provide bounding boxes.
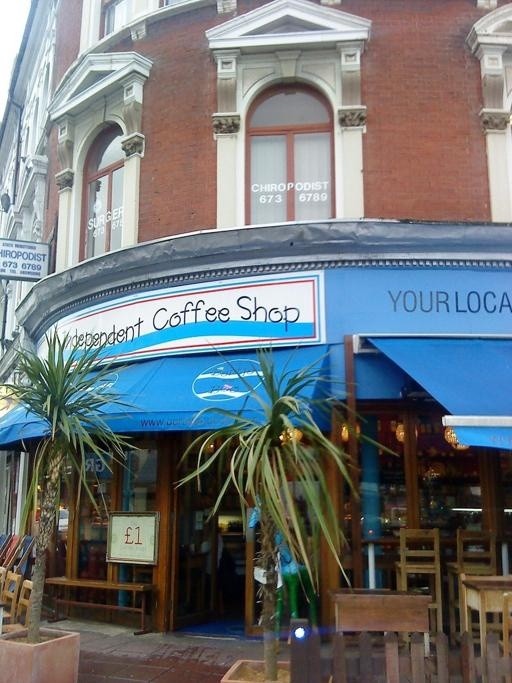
[189,515,224,611]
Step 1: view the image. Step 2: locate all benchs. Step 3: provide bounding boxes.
[45,575,158,635]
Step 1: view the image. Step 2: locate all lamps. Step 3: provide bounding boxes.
[276,419,471,450]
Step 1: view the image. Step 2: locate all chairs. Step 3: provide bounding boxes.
[393,524,512,683]
[1,565,34,633]
